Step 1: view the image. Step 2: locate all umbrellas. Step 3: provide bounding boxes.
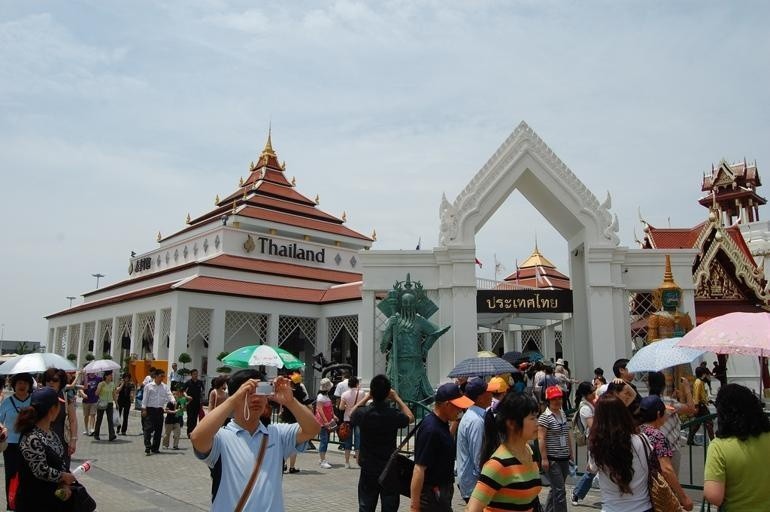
[625,337,708,373]
[671,312,770,400]
[0,353,77,374]
[221,345,305,370]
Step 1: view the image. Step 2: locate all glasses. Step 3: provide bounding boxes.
[590,387,596,395]
[46,377,60,383]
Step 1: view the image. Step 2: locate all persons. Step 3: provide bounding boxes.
[647,281,692,396]
[380,293,451,418]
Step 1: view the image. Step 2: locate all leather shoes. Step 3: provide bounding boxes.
[93,434,102,440]
[109,433,116,441]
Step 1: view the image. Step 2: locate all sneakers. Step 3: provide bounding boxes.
[144,446,179,456]
[571,490,578,506]
[319,458,332,469]
[344,461,350,469]
[283,463,300,473]
[83,428,95,436]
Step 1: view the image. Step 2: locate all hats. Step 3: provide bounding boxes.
[543,359,553,366]
[319,377,334,391]
[463,377,501,399]
[436,383,475,409]
[30,385,66,410]
[488,377,507,394]
[555,358,564,365]
[545,386,564,400]
[638,394,675,412]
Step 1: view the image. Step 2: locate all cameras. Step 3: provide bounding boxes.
[255,381,276,396]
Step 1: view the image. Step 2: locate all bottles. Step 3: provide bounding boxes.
[433,485,441,500]
[54,488,66,499]
[71,459,93,479]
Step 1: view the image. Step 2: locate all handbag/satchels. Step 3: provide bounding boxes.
[96,399,112,410]
[637,433,690,512]
[377,451,415,499]
[327,418,338,431]
[338,422,351,440]
[135,384,145,400]
[61,480,97,512]
